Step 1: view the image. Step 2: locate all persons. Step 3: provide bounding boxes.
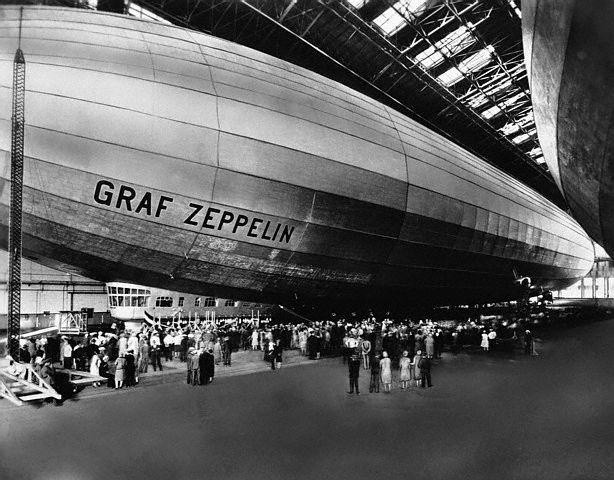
[19,317,292,394]
[292,310,546,394]
